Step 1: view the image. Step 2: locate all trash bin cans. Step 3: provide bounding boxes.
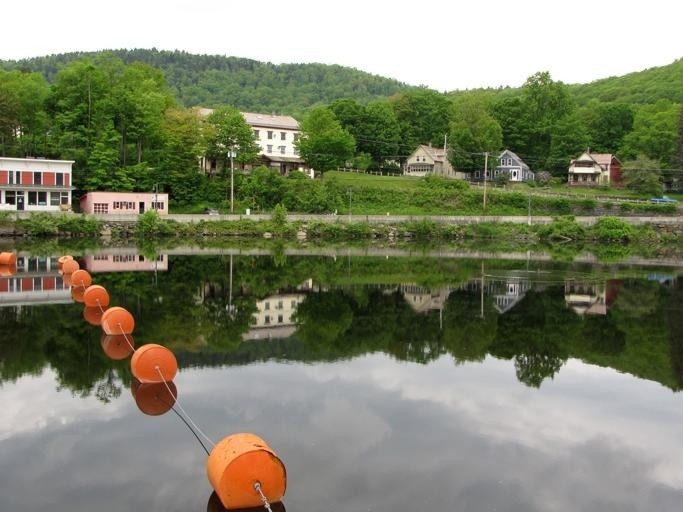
[246,208,251,215]
